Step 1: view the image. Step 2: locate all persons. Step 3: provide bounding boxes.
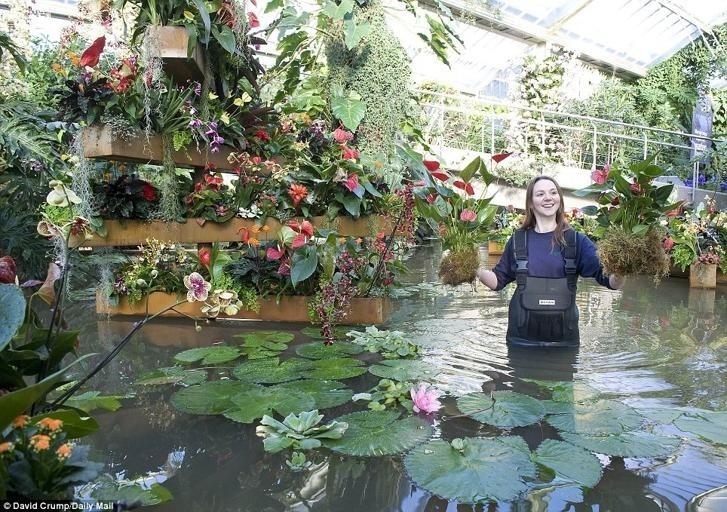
[475,176,626,347]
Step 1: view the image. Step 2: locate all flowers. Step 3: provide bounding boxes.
[489,147,725,292]
[49,1,430,332]
[411,146,516,292]
